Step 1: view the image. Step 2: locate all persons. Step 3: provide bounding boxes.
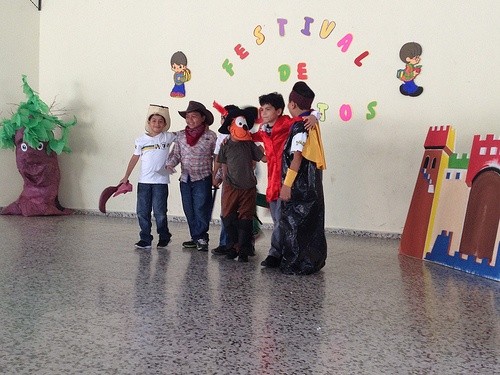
[120,104,178,249]
[165,101,217,250]
[210,81,328,275]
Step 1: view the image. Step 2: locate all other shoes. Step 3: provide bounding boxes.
[238,254,249,262]
[211,246,226,255]
[260,255,281,267]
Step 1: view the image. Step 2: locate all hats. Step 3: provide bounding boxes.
[177,100,214,127]
[144,103,172,133]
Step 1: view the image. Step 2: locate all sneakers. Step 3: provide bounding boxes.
[157,238,172,249]
[134,239,152,249]
[196,241,209,251]
[182,240,198,248]
